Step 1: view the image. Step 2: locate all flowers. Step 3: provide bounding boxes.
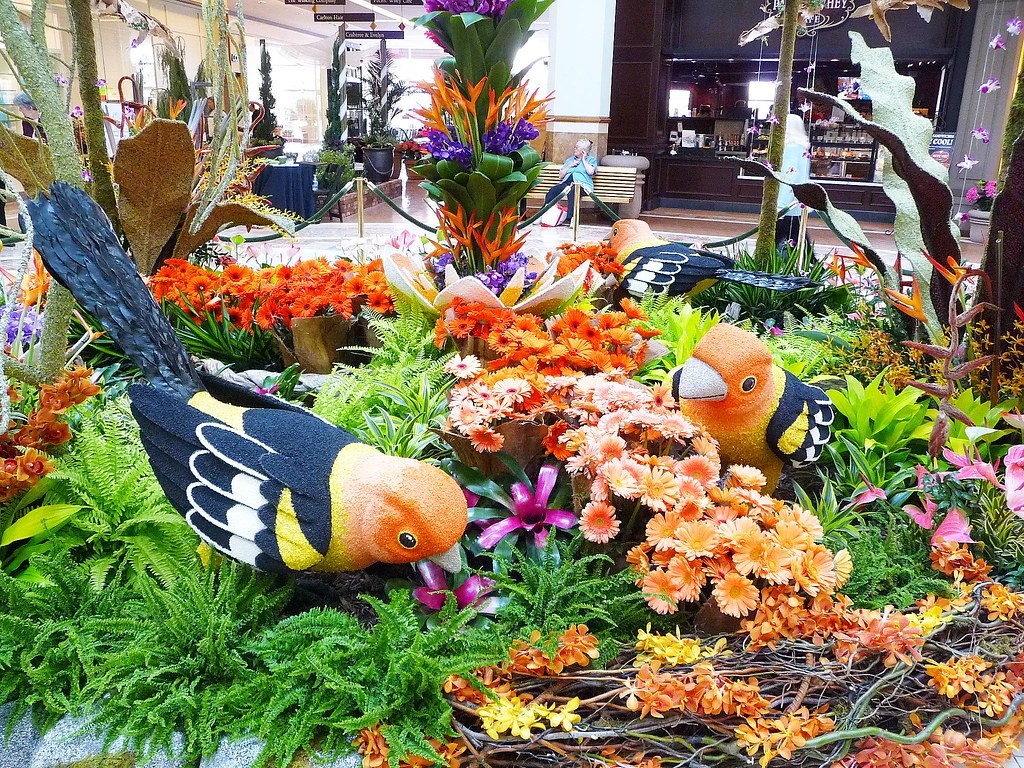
[402,141,429,161]
[966,180,996,212]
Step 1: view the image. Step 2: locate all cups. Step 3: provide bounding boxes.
[278,156,287,164]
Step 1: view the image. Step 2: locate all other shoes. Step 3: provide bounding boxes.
[569,217,573,228]
[533,216,542,224]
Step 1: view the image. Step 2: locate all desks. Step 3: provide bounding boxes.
[252,162,316,220]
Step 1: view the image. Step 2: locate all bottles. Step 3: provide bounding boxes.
[714,132,744,152]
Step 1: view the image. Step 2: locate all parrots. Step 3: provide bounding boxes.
[17,181,472,582]
[595,214,830,310]
[662,318,837,498]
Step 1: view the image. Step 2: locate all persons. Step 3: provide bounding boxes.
[774,113,825,275]
[533,138,597,228]
[206,96,227,144]
[249,104,259,123]
[0,89,48,247]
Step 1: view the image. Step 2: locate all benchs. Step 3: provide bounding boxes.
[520,165,636,227]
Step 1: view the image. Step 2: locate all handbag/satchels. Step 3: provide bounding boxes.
[540,202,568,227]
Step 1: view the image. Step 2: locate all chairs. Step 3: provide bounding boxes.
[316,163,348,222]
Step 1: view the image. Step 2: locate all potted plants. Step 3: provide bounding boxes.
[361,134,398,184]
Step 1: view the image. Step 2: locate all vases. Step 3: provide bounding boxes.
[968,210,991,243]
[403,160,425,180]
[392,152,401,179]
